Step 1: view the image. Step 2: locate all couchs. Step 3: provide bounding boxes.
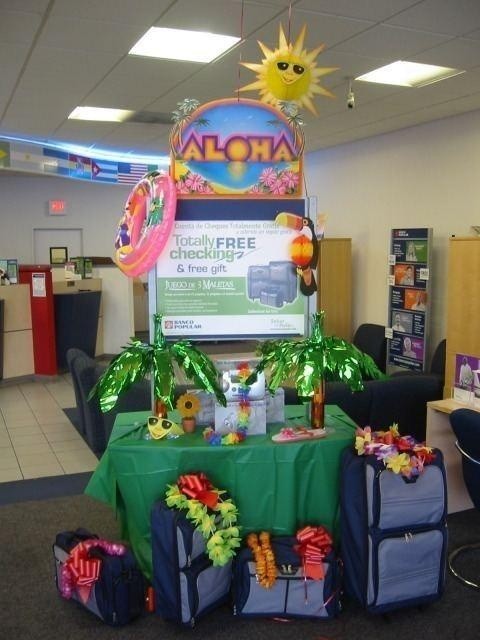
[65,324,444,452]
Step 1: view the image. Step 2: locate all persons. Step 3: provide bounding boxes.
[392,242,424,361]
[458,356,473,389]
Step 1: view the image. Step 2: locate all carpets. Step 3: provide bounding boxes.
[1,471,479,640]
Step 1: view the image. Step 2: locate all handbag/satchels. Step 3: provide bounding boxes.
[230,536,341,621]
[52,527,145,628]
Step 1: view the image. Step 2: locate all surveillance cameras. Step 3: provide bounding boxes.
[347,92,354,109]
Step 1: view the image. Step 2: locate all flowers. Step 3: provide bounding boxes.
[203,363,251,446]
[176,393,202,416]
[355,422,436,480]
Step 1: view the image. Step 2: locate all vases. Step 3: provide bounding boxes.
[182,417,196,433]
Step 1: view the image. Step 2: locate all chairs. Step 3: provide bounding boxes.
[447,408,479,591]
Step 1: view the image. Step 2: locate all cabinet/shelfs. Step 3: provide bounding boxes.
[444,237,480,398]
[0,283,36,381]
[319,238,353,339]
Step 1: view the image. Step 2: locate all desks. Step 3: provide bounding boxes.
[108,405,362,582]
[426,398,480,513]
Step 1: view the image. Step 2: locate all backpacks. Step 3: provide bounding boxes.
[151,488,238,629]
[340,435,449,613]
